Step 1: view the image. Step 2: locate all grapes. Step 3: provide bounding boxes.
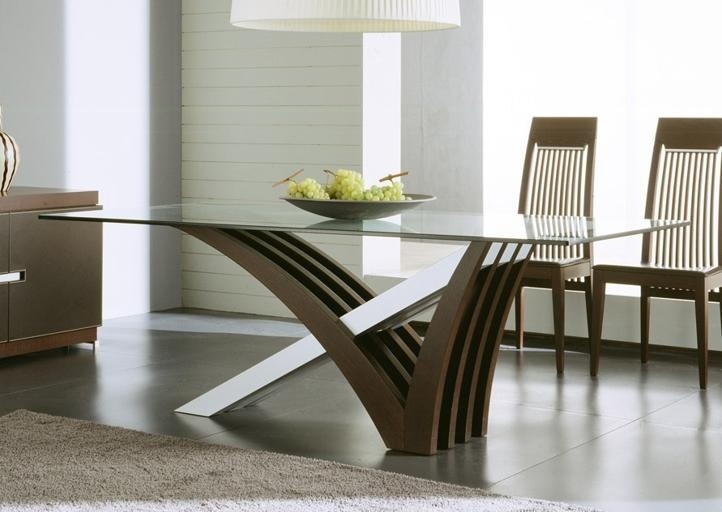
[357,171,412,200]
[272,168,330,200]
[323,169,364,200]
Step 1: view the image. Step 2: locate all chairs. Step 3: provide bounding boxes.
[515,117,596,378]
[589,118,721,389]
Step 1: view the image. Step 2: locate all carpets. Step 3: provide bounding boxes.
[0,410,595,512]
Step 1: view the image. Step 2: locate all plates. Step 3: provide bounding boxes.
[276,191,438,221]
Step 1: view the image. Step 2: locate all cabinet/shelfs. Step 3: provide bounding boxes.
[0,204,105,362]
